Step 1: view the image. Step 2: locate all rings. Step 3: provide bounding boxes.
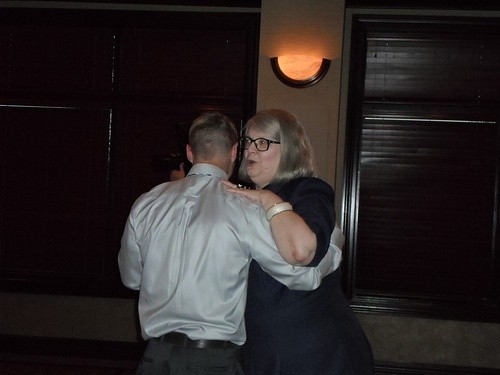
[237,184,242,188]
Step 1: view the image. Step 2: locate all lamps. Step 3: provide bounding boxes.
[261,3,345,88]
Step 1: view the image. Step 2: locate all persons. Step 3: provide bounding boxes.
[118,112,345,375]
[170,108,375,375]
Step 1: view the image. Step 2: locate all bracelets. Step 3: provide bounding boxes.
[266,201,292,221]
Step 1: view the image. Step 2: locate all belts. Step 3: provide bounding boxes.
[156,332,238,349]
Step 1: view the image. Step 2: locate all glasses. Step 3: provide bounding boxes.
[239,136,281,152]
